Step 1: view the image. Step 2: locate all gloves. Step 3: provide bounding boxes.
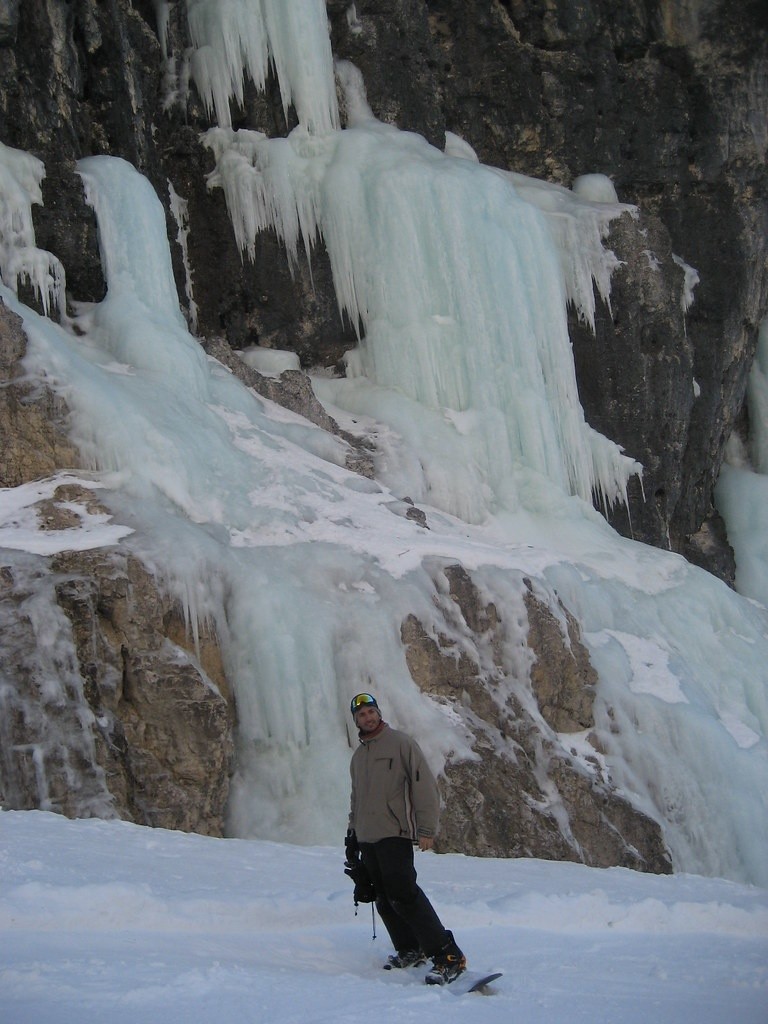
[344,829,360,862]
[343,861,378,889]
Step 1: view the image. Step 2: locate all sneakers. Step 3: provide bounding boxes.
[388,946,425,969]
[424,930,466,986]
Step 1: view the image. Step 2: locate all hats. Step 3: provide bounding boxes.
[350,693,378,714]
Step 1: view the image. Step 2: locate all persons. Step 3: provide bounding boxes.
[344,693,466,986]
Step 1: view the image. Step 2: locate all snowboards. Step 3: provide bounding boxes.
[380,953,505,996]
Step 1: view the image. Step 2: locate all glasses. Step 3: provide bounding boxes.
[353,695,373,709]
